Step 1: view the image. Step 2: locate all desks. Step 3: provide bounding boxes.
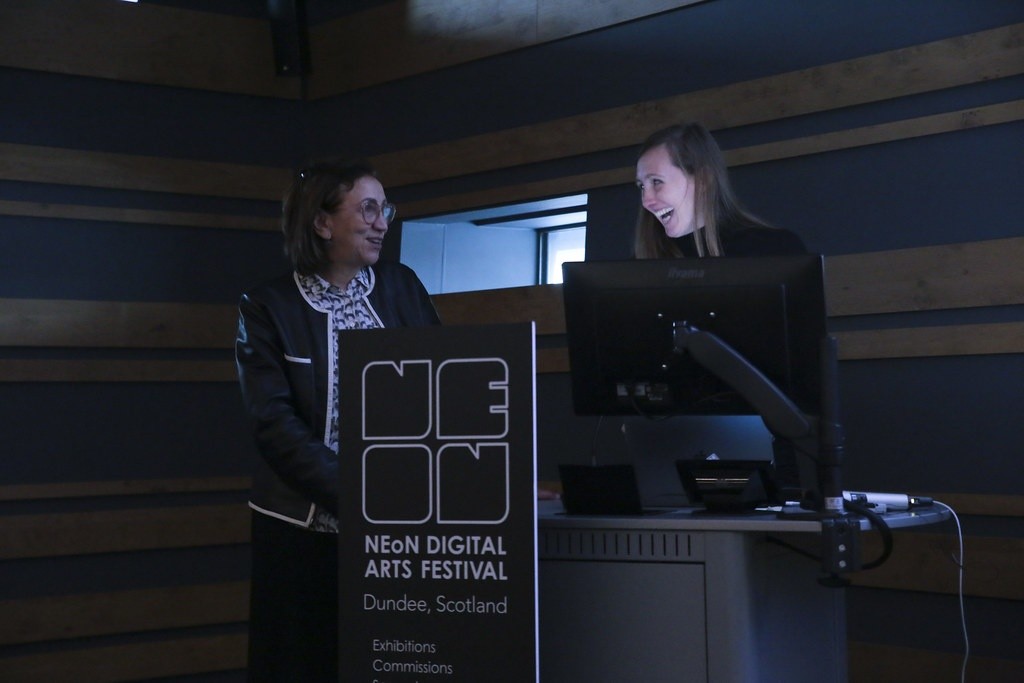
[537,488,953,683]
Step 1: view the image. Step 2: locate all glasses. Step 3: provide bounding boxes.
[330,198,397,226]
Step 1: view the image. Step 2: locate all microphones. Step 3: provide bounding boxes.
[841,489,934,511]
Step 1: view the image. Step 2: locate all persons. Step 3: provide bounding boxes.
[231,153,571,683]
[630,120,853,501]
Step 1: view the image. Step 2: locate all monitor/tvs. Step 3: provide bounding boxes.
[562,254,828,419]
